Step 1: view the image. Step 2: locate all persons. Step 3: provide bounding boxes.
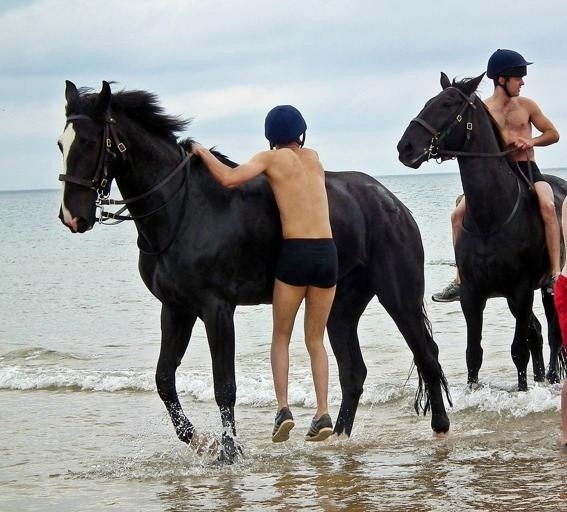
[181,102,339,441]
[431,47,559,301]
[553,193,567,449]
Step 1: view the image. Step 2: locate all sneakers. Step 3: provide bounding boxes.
[432,279,462,303]
[271,406,296,442]
[303,413,334,441]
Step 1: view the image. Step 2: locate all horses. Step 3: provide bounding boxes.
[58,80,453,467]
[397,71,567,396]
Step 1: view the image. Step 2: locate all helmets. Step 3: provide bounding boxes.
[486,48,534,75]
[264,104,308,145]
